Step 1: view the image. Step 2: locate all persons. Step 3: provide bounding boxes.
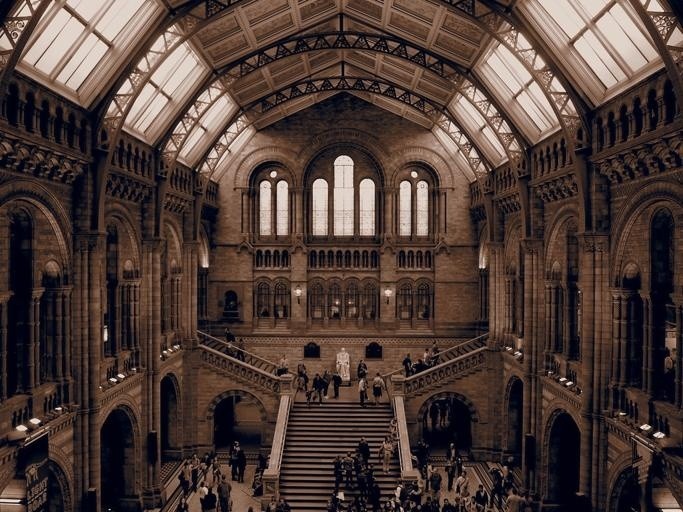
[328,418,533,511]
[175,441,293,512]
[224,326,452,430]
